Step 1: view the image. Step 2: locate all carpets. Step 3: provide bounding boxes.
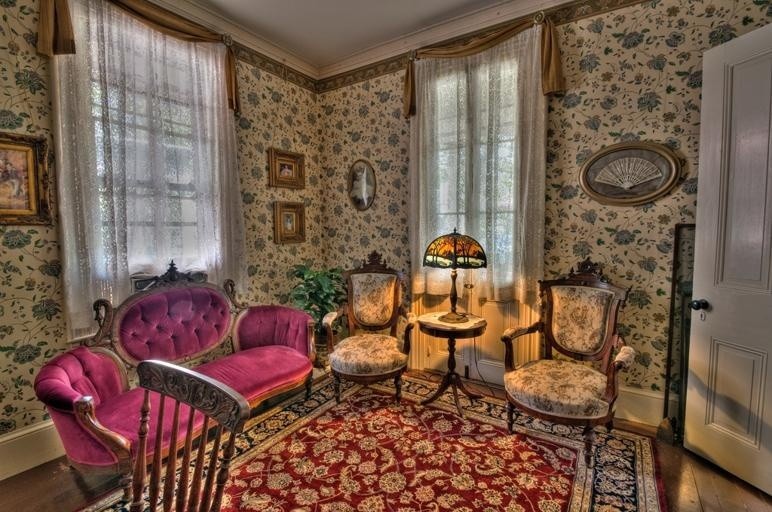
[75,365,673,511]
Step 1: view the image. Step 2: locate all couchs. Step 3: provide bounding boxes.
[35,260,316,503]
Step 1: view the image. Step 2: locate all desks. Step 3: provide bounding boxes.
[417,314,487,418]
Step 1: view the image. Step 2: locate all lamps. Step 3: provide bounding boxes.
[423,226,490,325]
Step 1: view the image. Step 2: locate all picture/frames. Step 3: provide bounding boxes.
[267,148,307,242]
[348,159,376,211]
[0,132,53,226]
[579,141,682,207]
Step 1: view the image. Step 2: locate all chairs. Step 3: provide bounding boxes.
[321,251,416,404]
[126,360,250,511]
[501,257,635,467]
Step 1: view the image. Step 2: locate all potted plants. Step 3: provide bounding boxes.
[285,264,349,367]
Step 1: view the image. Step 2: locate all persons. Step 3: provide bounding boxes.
[2,163,20,196]
